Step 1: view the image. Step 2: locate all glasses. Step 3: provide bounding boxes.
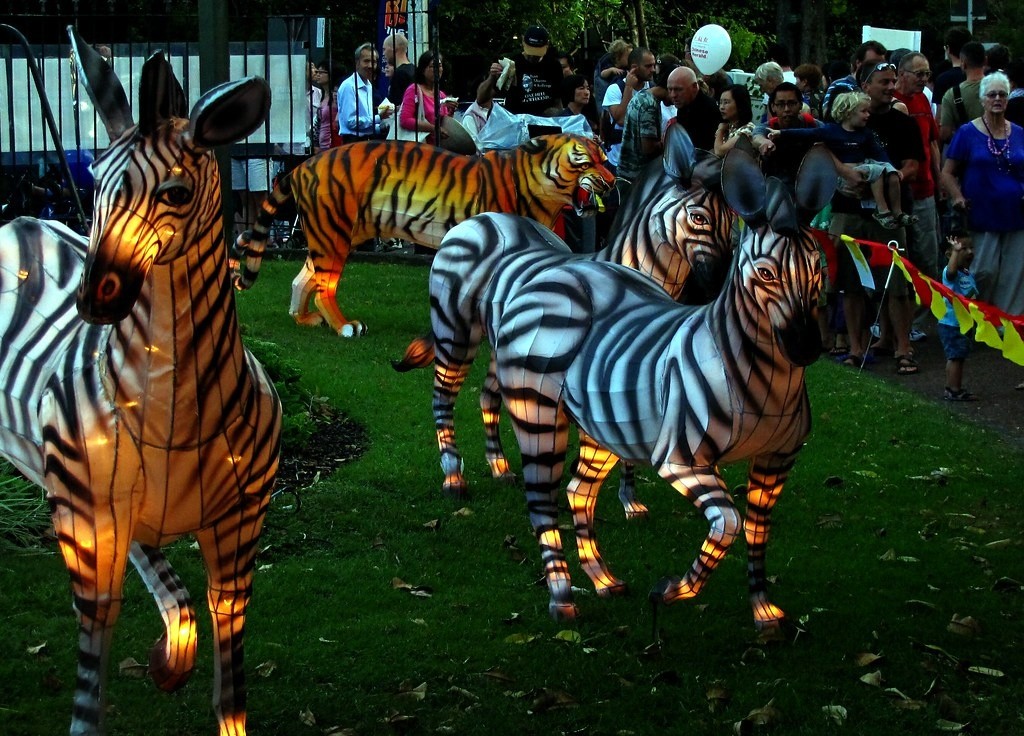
[773,99,798,109]
[943,45,948,51]
[864,62,896,83]
[315,70,328,74]
[903,68,932,78]
[986,90,1008,99]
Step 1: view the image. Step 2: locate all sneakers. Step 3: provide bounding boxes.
[869,323,882,338]
[908,328,927,341]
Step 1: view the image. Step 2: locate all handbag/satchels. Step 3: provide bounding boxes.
[387,84,431,142]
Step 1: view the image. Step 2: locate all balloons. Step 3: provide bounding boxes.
[690,23,732,76]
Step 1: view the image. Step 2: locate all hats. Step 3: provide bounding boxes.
[524,26,550,56]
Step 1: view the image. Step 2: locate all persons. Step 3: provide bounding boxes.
[0,44,307,248]
[307,26,1024,399]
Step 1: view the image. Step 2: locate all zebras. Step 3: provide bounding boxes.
[390,119,737,496]
[0,21,282,736]
[494,143,839,636]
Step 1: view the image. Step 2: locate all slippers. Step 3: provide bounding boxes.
[894,347,920,375]
[841,355,864,368]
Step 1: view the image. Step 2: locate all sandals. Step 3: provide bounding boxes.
[872,205,900,229]
[893,212,919,225]
[944,386,977,401]
[828,344,848,354]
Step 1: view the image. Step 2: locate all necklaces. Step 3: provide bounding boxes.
[983,114,1011,176]
